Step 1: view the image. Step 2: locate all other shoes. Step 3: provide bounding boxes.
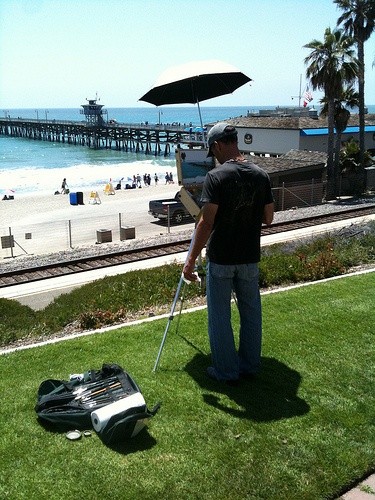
[240,372,256,379]
[207,366,240,384]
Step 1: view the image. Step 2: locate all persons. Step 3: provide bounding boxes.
[183,122,275,387]
[140,120,193,131]
[61,177,68,195]
[109,172,176,195]
[3,195,7,200]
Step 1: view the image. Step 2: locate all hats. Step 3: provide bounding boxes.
[206,122,237,158]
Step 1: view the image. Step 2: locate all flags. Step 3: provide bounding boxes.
[303,83,314,108]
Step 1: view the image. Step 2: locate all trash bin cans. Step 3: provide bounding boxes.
[70,192,83,205]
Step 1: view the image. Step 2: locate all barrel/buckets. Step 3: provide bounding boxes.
[70,193,77,205]
[76,192,83,204]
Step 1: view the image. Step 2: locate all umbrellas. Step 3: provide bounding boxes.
[136,57,253,149]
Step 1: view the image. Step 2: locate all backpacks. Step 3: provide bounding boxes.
[34,363,162,455]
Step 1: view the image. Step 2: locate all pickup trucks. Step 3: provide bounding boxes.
[148,191,191,224]
